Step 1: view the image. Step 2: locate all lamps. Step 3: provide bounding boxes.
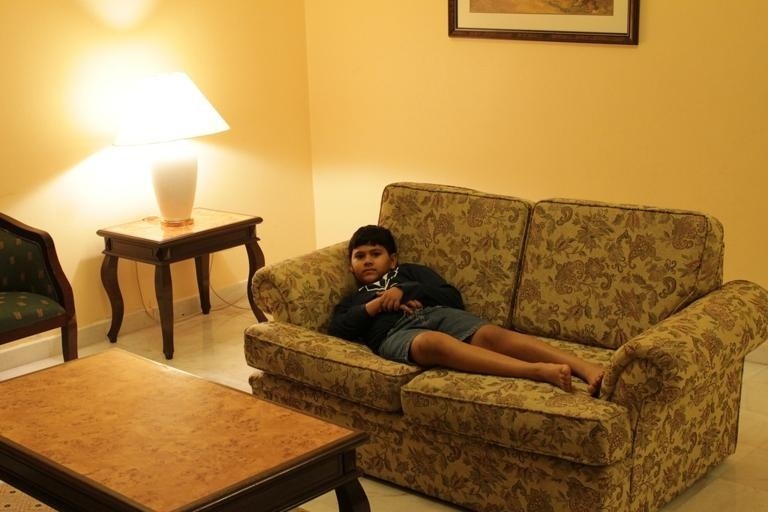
[114,68,230,230]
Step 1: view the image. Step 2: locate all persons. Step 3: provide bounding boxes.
[325,225,608,399]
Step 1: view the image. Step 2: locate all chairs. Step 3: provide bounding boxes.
[0,213,77,382]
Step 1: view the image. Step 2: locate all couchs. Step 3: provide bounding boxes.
[243,181,767,511]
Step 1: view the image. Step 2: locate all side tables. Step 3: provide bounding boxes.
[96,208,268,360]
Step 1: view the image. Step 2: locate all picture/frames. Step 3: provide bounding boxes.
[448,0,640,46]
